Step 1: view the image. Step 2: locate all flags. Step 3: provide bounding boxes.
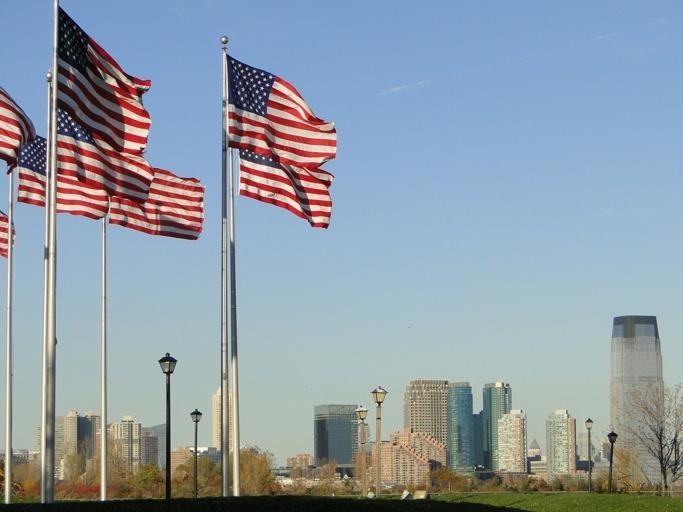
[56,109,155,205]
[16,134,109,221]
[1,86,36,174]
[222,53,337,166]
[109,168,205,240]
[56,5,152,159]
[233,146,335,231]
[0,211,16,259]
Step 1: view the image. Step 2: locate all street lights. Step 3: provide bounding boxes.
[190,408,203,499]
[369,385,389,498]
[354,404,369,496]
[585,418,594,493]
[158,352,178,499]
[607,430,618,494]
